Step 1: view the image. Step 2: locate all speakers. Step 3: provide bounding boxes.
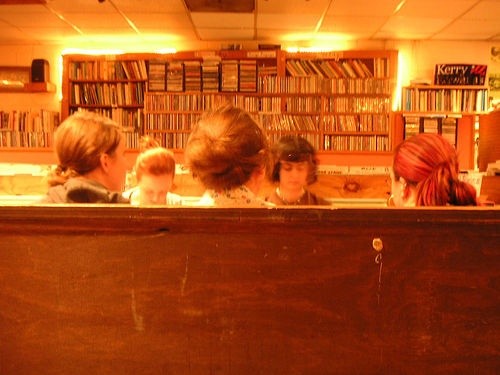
[31,59,49,81]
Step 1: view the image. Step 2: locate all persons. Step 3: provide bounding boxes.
[121,136,184,204]
[393,134,477,206]
[38,111,130,203]
[264,135,332,205]
[184,106,275,206]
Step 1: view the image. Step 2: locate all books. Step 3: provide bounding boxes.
[150,59,277,92]
[146,96,389,151]
[403,88,488,112]
[71,83,144,105]
[0,109,59,148]
[69,61,147,80]
[405,118,456,144]
[257,58,388,94]
[69,107,141,147]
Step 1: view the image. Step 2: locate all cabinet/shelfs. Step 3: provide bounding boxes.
[0,48,489,167]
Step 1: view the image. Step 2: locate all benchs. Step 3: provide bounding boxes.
[0,203,500,375]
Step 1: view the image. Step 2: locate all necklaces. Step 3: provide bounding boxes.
[276,189,305,205]
